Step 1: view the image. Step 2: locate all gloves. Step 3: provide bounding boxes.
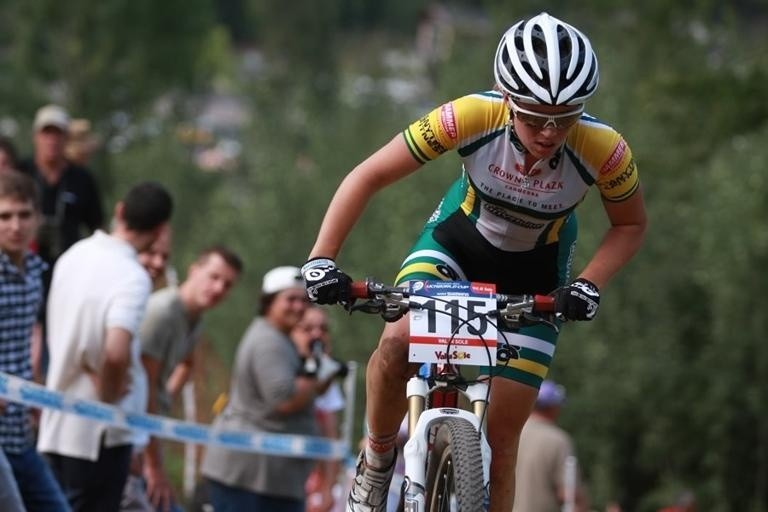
[548,275,601,323]
[299,255,355,307]
[311,349,348,397]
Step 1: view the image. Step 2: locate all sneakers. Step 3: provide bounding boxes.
[344,441,399,512]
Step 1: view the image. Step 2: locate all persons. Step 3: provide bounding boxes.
[301,9,651,511]
[0,100,347,510]
[514,381,579,512]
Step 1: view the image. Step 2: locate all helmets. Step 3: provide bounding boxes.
[493,9,601,108]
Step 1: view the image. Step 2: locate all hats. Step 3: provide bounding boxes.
[535,377,569,408]
[31,102,72,136]
[259,265,309,298]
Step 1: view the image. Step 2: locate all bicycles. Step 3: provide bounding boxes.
[337,277,573,512]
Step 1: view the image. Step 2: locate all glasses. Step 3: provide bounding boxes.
[507,94,585,131]
[0,208,36,222]
